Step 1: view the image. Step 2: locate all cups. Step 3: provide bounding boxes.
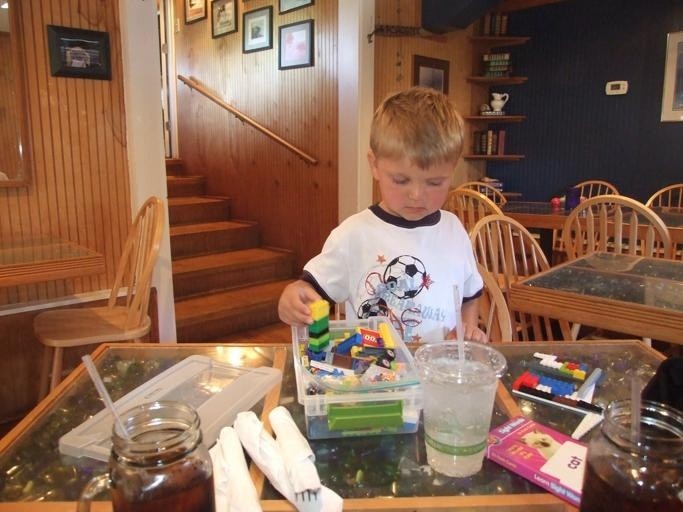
[564,186,581,209]
[412,338,508,478]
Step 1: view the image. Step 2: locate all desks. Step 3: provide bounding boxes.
[509,250,682,350]
[454,207,681,265]
[0,236,107,292]
[0,329,677,511]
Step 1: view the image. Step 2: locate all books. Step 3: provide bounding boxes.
[479,111,505,116]
[484,415,589,508]
[477,179,503,193]
[472,129,506,156]
[480,12,514,79]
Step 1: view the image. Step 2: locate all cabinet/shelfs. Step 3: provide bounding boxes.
[460,30,532,205]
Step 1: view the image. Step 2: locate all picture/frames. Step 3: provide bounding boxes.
[209,0,238,41]
[276,0,315,16]
[42,23,112,81]
[411,53,450,100]
[183,0,207,25]
[240,5,273,54]
[276,17,314,72]
[657,30,682,124]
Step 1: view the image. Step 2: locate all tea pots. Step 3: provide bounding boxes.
[490,92,509,111]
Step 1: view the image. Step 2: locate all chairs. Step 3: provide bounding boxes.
[442,187,503,234]
[469,215,573,343]
[563,194,673,261]
[32,193,163,410]
[575,179,620,199]
[463,181,507,203]
[646,183,683,208]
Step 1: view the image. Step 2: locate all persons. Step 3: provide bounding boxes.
[278,85,490,347]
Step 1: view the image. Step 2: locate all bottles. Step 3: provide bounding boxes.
[572,399,682,511]
[106,400,216,512]
